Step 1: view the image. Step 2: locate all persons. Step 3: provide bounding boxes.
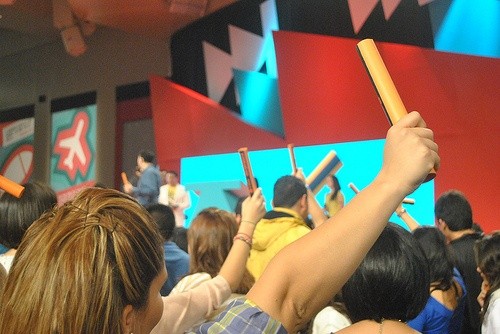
[322,175,344,218]
[0,109,500,334]
[158,171,191,228]
[123,150,160,210]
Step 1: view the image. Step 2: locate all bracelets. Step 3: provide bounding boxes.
[241,217,257,227]
[232,232,253,249]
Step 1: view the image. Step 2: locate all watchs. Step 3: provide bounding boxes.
[397,208,406,217]
